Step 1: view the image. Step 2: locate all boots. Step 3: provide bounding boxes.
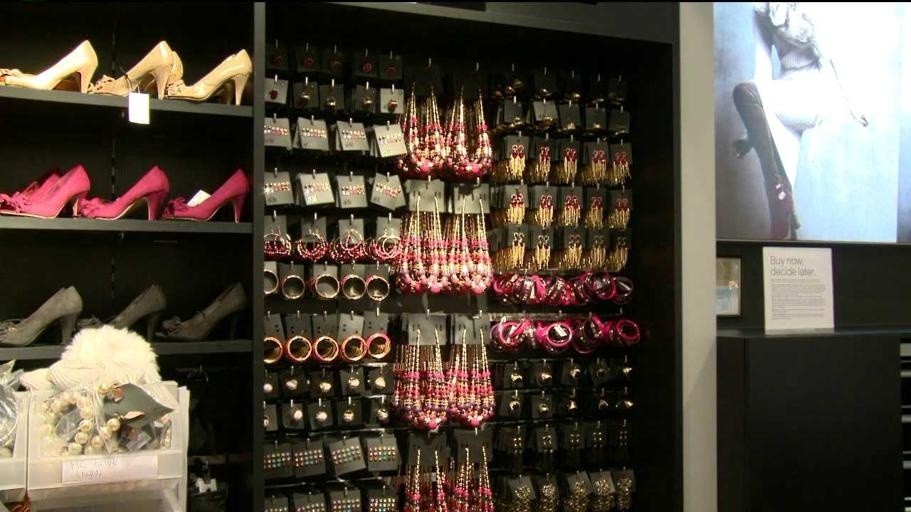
[731,83,801,240]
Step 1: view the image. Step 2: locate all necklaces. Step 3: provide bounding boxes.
[394,446,494,512]
[393,191,494,296]
[393,81,495,178]
[392,325,495,437]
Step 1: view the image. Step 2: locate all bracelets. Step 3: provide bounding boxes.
[489,274,641,357]
[262,226,399,364]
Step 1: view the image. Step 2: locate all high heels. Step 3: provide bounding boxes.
[165,48,252,106]
[161,167,249,223]
[87,40,174,99]
[133,50,182,98]
[162,280,247,342]
[223,54,235,104]
[77,164,164,221]
[78,283,167,343]
[0,39,98,93]
[0,286,82,346]
[0,164,90,219]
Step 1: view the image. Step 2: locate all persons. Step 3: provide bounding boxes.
[733,1,870,239]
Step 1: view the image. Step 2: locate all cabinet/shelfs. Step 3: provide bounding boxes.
[0,0,260,510]
[262,4,681,511]
